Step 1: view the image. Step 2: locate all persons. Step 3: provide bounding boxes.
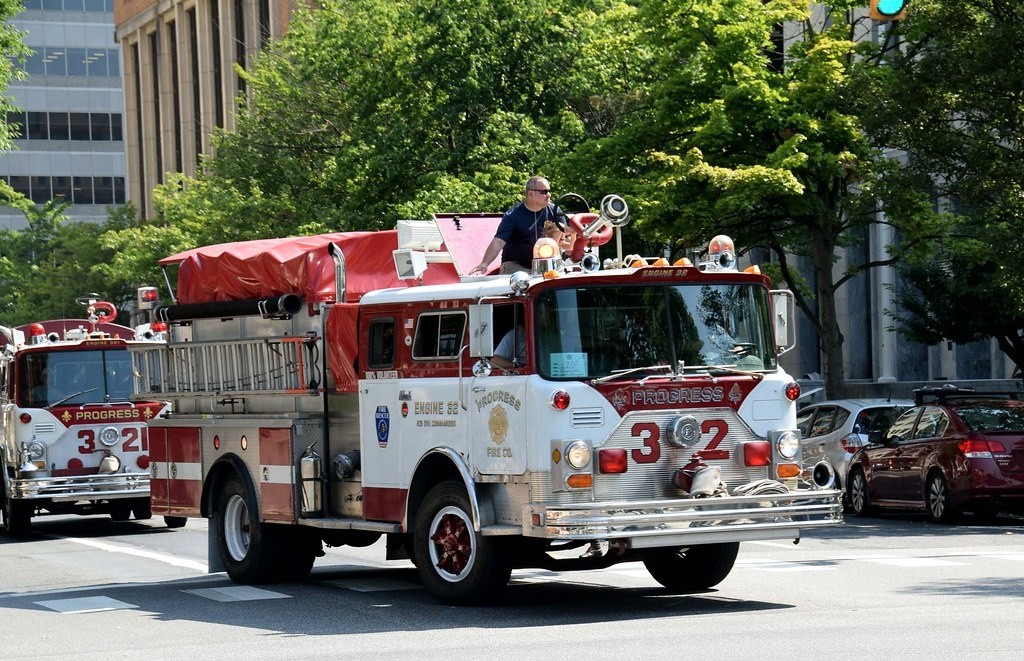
[467,174,575,274]
[493,301,564,376]
[32,368,62,401]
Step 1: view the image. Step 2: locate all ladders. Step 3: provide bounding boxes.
[125,329,326,401]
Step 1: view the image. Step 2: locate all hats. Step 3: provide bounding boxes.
[41,368,55,376]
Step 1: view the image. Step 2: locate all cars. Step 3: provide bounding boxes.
[797,400,924,509]
[844,384,1023,523]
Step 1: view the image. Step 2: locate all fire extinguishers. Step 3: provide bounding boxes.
[298,441,326,519]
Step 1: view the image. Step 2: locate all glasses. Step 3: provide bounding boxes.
[529,189,550,194]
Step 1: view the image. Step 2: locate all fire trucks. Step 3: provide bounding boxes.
[131,194,846,610]
[0,298,188,538]
